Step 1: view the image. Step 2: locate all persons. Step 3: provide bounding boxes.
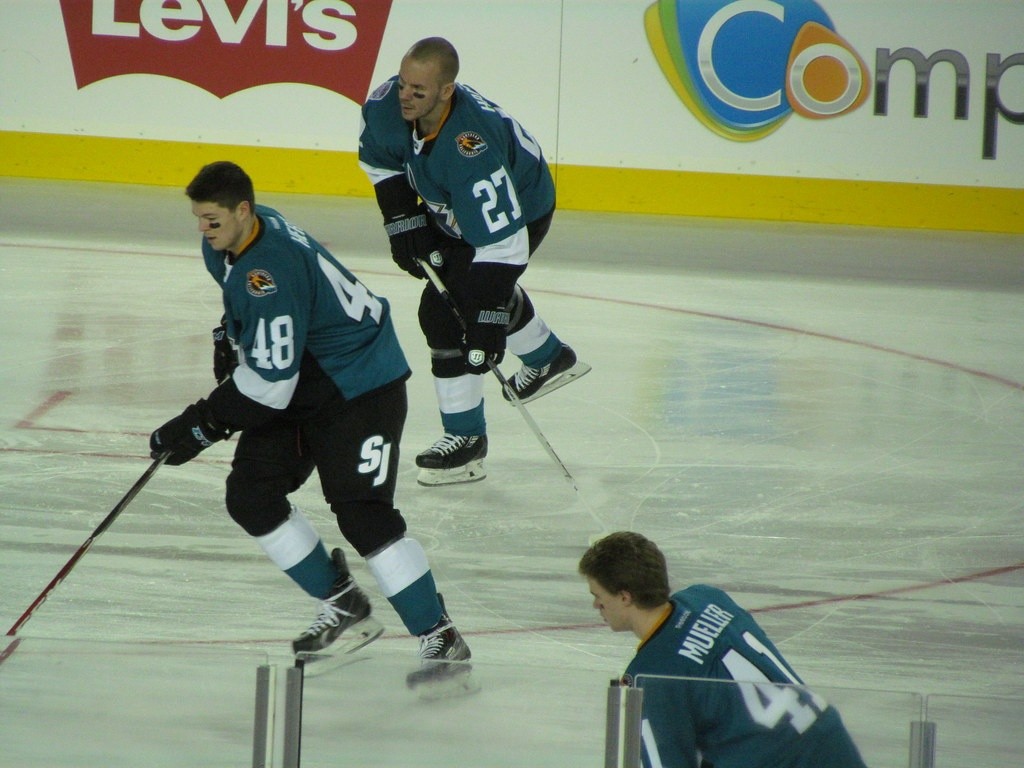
[357,37,592,486]
[580,531,868,768]
[150,162,480,701]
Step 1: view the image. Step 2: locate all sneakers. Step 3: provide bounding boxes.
[502,341,592,407]
[293,549,385,664]
[416,430,488,487]
[406,593,482,703]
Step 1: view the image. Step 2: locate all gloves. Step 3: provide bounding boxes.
[212,321,243,385]
[374,201,449,280]
[149,398,235,465]
[448,282,514,376]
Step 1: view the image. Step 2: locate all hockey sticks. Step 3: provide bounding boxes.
[1,448,176,666]
[416,254,612,548]
